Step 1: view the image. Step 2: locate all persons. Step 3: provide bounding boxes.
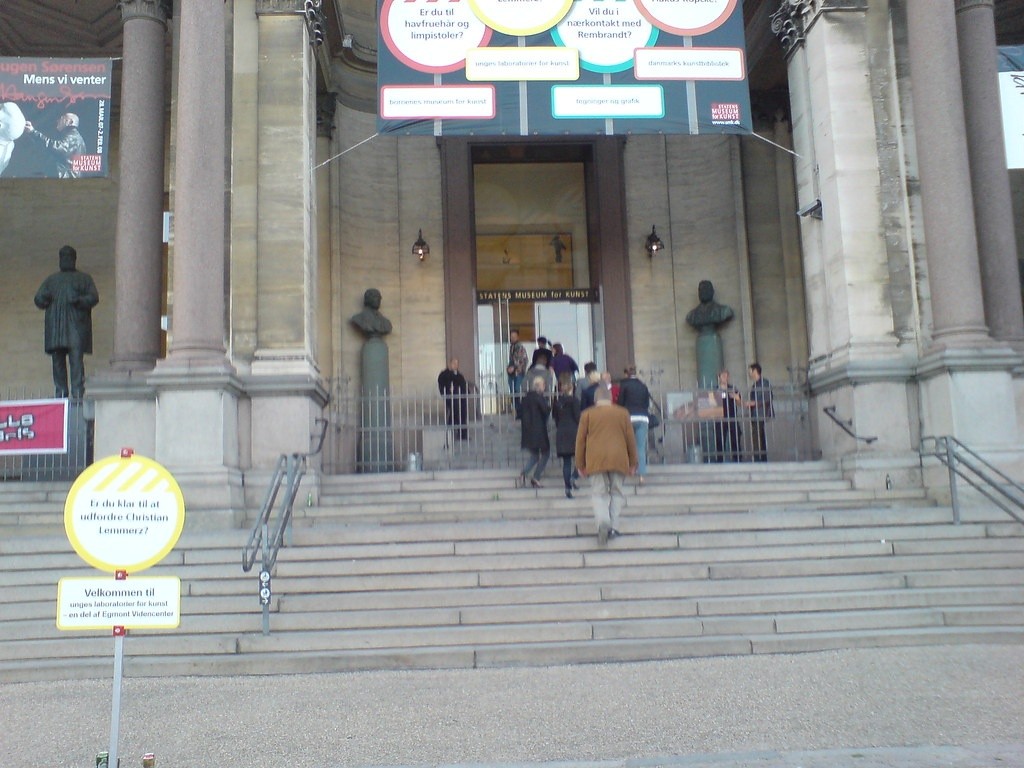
[552,383,583,498]
[575,362,597,400]
[437,358,470,439]
[25,113,86,178]
[34,245,99,399]
[522,354,553,401]
[552,371,575,427]
[732,363,776,462]
[351,290,392,335]
[713,369,742,463]
[528,337,551,370]
[550,344,579,385]
[514,376,550,487]
[618,365,649,486]
[580,371,601,411]
[685,279,733,332]
[575,385,638,545]
[507,329,528,419]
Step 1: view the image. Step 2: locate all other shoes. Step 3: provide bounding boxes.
[565,488,573,499]
[639,476,645,487]
[608,528,619,538]
[572,469,579,479]
[571,477,579,490]
[598,524,608,546]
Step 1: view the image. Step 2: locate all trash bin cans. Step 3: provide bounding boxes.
[403,451,422,470]
[687,443,703,463]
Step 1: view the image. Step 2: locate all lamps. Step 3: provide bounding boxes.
[412,229,430,261]
[645,223,665,257]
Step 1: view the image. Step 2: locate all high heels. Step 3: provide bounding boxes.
[520,472,526,485]
[531,478,544,488]
